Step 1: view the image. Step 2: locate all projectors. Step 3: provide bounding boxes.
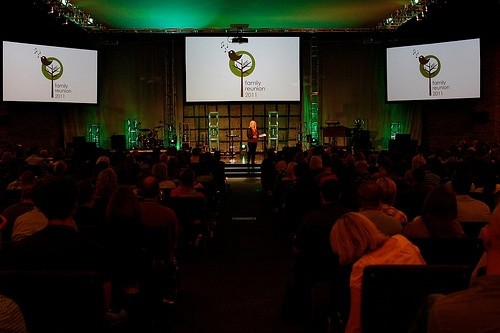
[232,38,248,43]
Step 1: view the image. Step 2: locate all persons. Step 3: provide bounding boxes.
[246,120,259,166]
[7,175,111,333]
[0,144,225,247]
[329,212,427,333]
[292,179,357,322]
[258,136,500,239]
[410,185,483,264]
[427,202,500,333]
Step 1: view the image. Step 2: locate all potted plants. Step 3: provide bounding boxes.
[351,116,369,131]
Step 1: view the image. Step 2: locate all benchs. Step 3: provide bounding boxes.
[362,265,486,333]
